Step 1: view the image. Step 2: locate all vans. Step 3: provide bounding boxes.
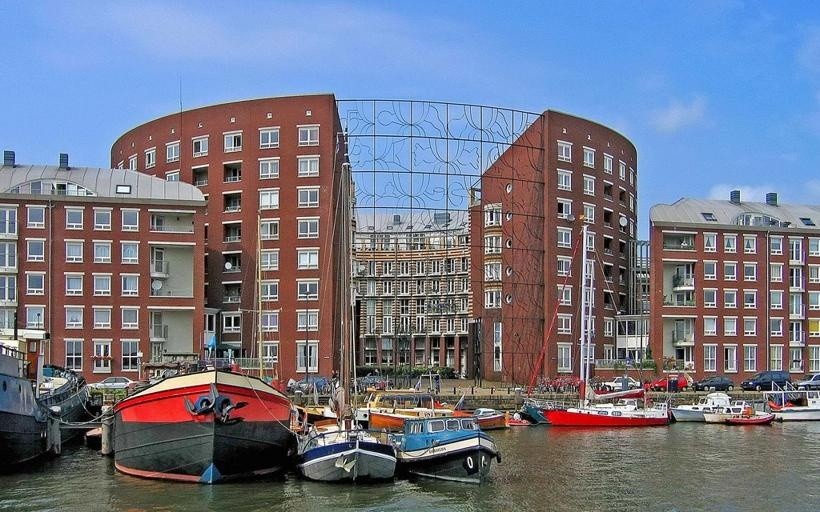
[741,371,791,391]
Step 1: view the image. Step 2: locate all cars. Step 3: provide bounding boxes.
[691,376,734,391]
[87,377,138,390]
[596,376,642,391]
[643,375,688,392]
[793,374,820,390]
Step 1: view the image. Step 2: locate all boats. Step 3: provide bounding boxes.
[302,384,508,484]
[0,344,87,469]
[112,370,293,484]
[671,394,820,426]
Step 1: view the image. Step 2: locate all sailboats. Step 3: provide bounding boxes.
[515,223,669,428]
[297,163,395,483]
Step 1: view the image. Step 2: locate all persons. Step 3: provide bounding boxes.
[388,380,394,390]
[416,376,419,391]
[680,241,687,248]
[375,378,387,390]
[433,373,439,391]
